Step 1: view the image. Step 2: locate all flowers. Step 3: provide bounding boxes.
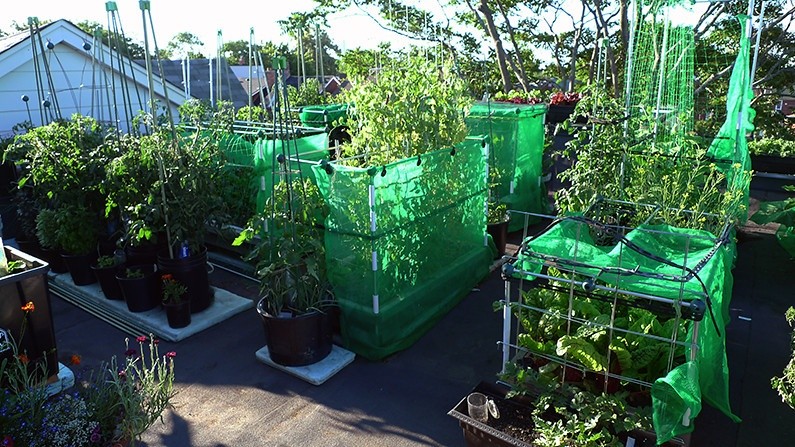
[489,89,580,106]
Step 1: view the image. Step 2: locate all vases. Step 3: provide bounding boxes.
[546,104,588,123]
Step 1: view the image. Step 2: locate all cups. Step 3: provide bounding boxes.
[467,393,488,423]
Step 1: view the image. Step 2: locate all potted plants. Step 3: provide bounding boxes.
[485,158,511,257]
[520,263,687,406]
[0,99,338,368]
[748,136,795,175]
